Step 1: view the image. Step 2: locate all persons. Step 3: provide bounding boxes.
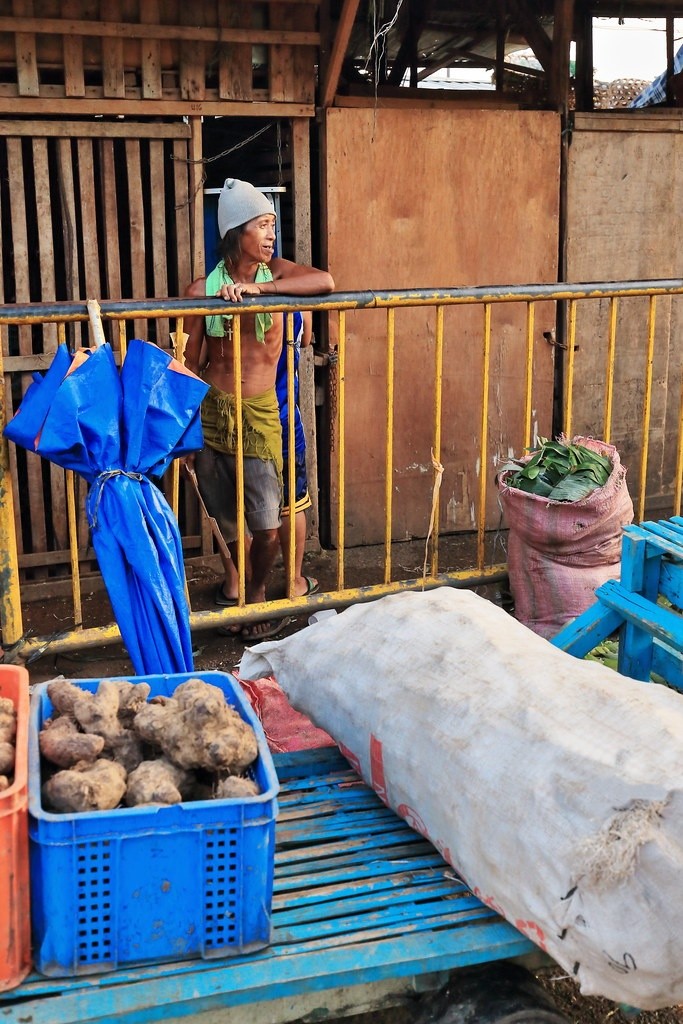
[181,177,335,640]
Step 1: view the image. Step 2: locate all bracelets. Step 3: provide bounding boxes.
[270,281,277,294]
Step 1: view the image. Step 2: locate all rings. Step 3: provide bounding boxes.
[238,287,242,291]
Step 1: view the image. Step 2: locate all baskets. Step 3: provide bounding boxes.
[1,661,281,996]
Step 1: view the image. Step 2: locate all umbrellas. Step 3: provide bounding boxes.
[2,299,211,676]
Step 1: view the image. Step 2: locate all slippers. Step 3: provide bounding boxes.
[216,621,240,637]
[301,575,320,598]
[216,580,238,606]
[241,616,291,640]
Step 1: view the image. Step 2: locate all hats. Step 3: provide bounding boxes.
[217,178,277,239]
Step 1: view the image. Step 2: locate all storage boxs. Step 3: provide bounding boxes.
[32,669,284,982]
[0,663,32,993]
